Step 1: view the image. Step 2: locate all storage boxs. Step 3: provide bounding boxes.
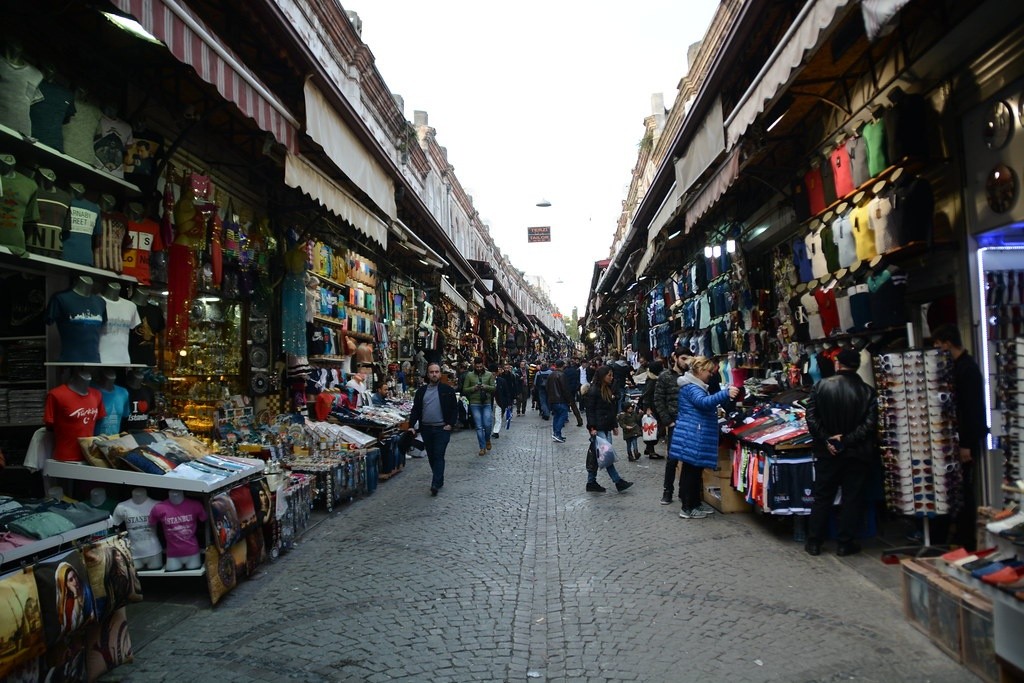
[702,460,754,513]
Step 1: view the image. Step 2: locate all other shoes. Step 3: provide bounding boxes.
[492,433,499,438]
[431,480,444,494]
[577,423,583,426]
[616,479,633,492]
[551,434,566,442]
[649,453,663,459]
[542,415,550,420]
[517,411,520,416]
[805,541,820,556]
[644,450,650,455]
[405,453,412,459]
[479,449,486,455]
[485,438,491,450]
[585,481,606,492]
[836,539,853,556]
[522,411,525,415]
[940,479,1023,605]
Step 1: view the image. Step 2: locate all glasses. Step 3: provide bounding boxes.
[870,348,965,517]
[993,336,1024,511]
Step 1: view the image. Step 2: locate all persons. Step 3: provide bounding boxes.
[43,369,156,462]
[0,36,133,179]
[488,357,583,443]
[654,346,715,514]
[124,141,158,184]
[455,357,496,456]
[209,207,240,250]
[167,182,205,351]
[55,562,97,643]
[678,239,737,357]
[804,325,991,556]
[408,362,457,495]
[0,153,165,285]
[44,275,166,367]
[104,544,142,613]
[371,381,388,404]
[78,488,208,570]
[579,350,668,495]
[286,226,346,284]
[787,85,933,386]
[669,355,740,519]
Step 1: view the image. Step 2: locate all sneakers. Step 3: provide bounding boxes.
[694,504,714,513]
[679,507,707,518]
[660,489,672,504]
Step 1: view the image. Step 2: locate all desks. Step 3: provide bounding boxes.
[899,558,1016,683]
[306,269,346,362]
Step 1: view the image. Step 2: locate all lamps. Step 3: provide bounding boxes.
[536,199,551,207]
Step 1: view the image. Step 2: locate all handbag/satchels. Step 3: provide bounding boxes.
[398,430,416,455]
[641,413,657,441]
[585,435,620,472]
[636,382,657,411]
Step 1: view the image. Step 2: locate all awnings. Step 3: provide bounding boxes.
[723,0,853,152]
[233,0,490,297]
[111,0,301,156]
[284,152,389,251]
[386,217,449,269]
[526,314,559,338]
[466,259,534,328]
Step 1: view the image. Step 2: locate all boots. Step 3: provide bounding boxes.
[628,454,637,461]
[634,448,641,460]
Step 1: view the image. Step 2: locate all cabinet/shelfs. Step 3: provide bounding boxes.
[0,335,47,427]
[0,122,149,368]
